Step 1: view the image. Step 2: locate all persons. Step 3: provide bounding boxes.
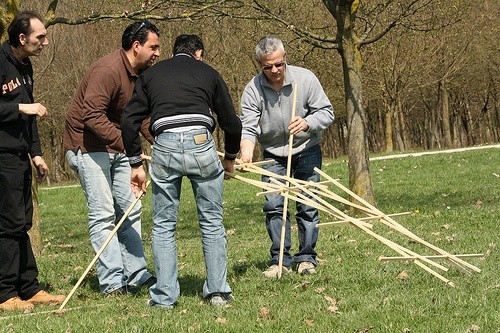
[0,10,65,314]
[120,34,242,309]
[63,18,161,294]
[237,35,335,279]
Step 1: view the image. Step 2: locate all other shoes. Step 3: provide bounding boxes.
[104,285,137,299]
[0,297,35,312]
[28,291,67,306]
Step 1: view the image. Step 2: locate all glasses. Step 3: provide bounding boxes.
[258,56,286,70]
[130,20,149,41]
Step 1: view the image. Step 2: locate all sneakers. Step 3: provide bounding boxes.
[295,260,317,275]
[210,293,236,305]
[262,265,293,279]
[148,299,176,308]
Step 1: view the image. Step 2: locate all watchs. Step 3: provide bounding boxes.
[304,123,309,132]
